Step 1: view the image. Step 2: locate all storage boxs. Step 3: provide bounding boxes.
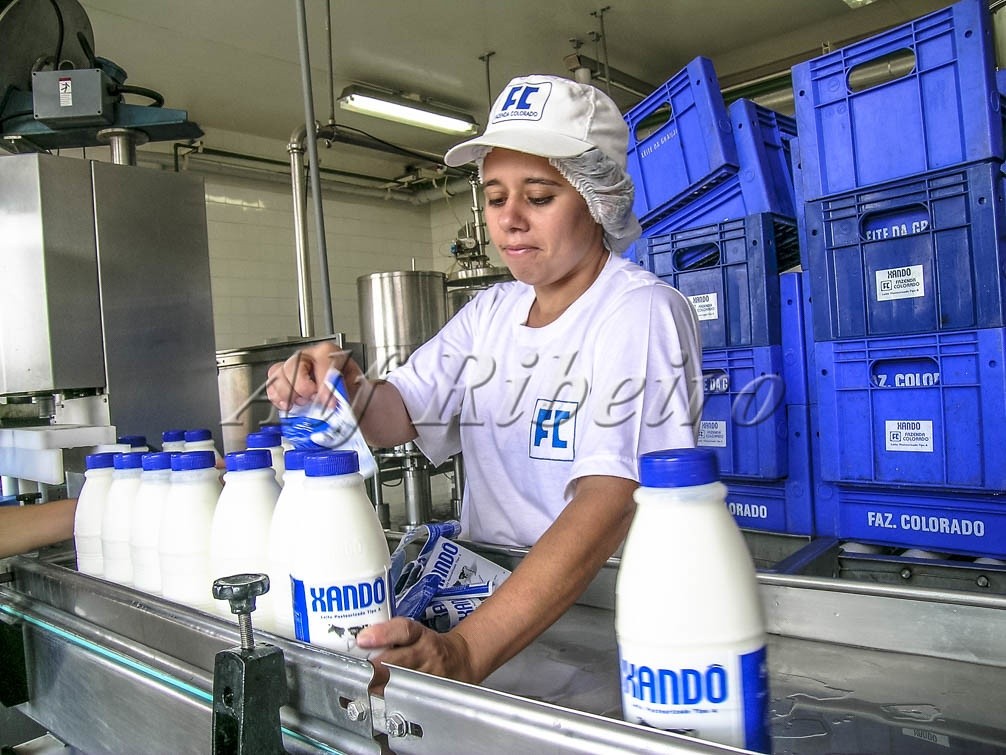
[699,343,788,482]
[790,0,1006,202]
[800,159,1006,340]
[635,214,794,346]
[818,327,1006,493]
[639,97,796,238]
[625,54,738,218]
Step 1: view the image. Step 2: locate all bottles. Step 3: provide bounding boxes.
[615,445,773,754]
[72,425,397,661]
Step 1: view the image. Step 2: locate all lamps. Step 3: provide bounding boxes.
[335,85,479,139]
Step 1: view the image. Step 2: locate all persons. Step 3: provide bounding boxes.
[0,498,79,559]
[262,76,708,685]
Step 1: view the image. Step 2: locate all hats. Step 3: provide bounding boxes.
[444,76,629,167]
